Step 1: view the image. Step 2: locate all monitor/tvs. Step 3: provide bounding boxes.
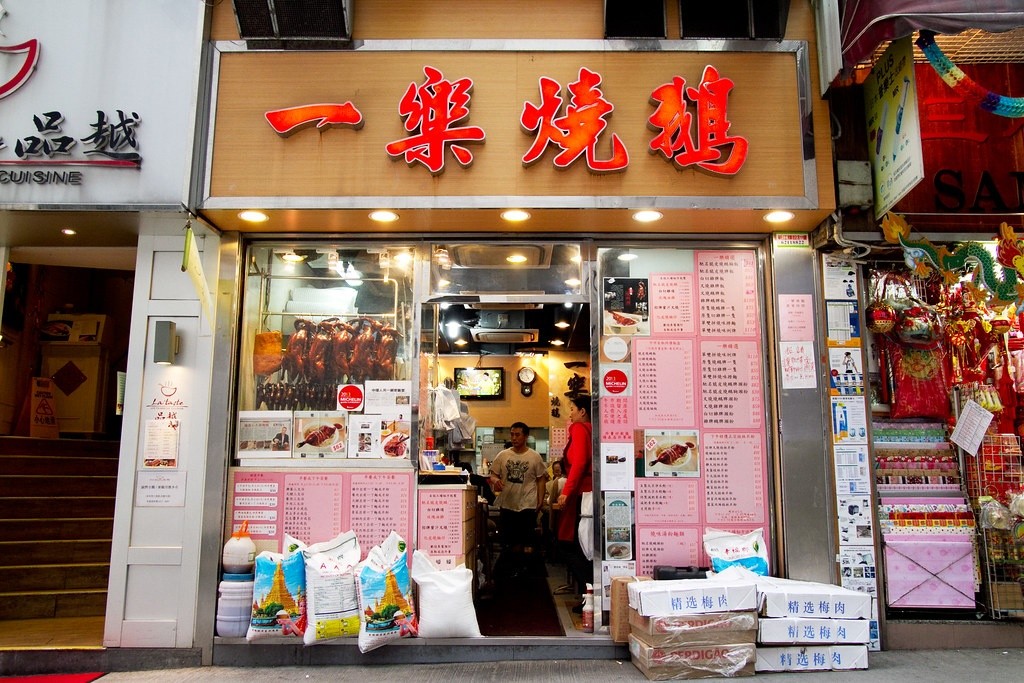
[454,367,505,400]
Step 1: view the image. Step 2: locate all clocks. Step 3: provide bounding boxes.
[517,367,536,397]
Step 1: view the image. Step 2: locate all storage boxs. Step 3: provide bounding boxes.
[609,569,871,682]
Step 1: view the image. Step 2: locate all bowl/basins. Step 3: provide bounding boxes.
[301,421,340,448]
[607,310,643,333]
[277,611,287,619]
[394,611,403,620]
[652,438,691,469]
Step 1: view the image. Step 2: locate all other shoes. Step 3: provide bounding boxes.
[571,604,584,614]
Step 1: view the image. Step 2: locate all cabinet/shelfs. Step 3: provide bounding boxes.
[416,483,478,605]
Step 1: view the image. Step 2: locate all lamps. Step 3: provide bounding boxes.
[153,320,179,365]
[547,267,583,346]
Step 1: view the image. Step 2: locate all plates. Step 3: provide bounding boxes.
[685,441,696,449]
[607,543,631,559]
[381,429,391,436]
[377,433,410,462]
[333,422,343,431]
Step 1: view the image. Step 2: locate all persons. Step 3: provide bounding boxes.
[842,352,858,373]
[456,462,497,564]
[545,461,567,505]
[487,421,546,594]
[553,394,592,613]
[549,458,568,532]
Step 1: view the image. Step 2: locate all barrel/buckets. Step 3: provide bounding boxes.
[219,519,256,575]
[216,573,253,637]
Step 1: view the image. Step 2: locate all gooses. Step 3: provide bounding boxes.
[256,316,405,412]
[648,443,689,468]
